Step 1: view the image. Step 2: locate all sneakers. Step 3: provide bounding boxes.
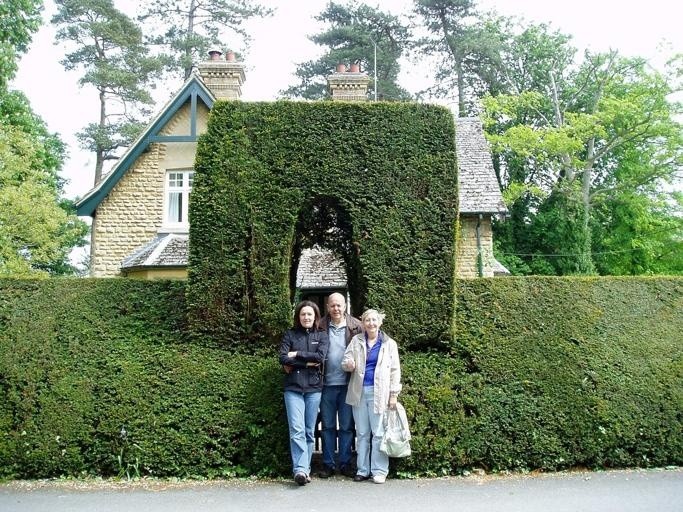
[354,475,386,483]
[294,472,311,486]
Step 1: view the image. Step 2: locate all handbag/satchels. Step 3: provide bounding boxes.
[380,429,412,457]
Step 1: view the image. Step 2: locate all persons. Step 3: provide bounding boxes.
[318,290,363,479]
[278,299,330,487]
[339,307,402,484]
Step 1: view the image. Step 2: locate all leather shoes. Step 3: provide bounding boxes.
[340,465,357,477]
[319,465,334,478]
